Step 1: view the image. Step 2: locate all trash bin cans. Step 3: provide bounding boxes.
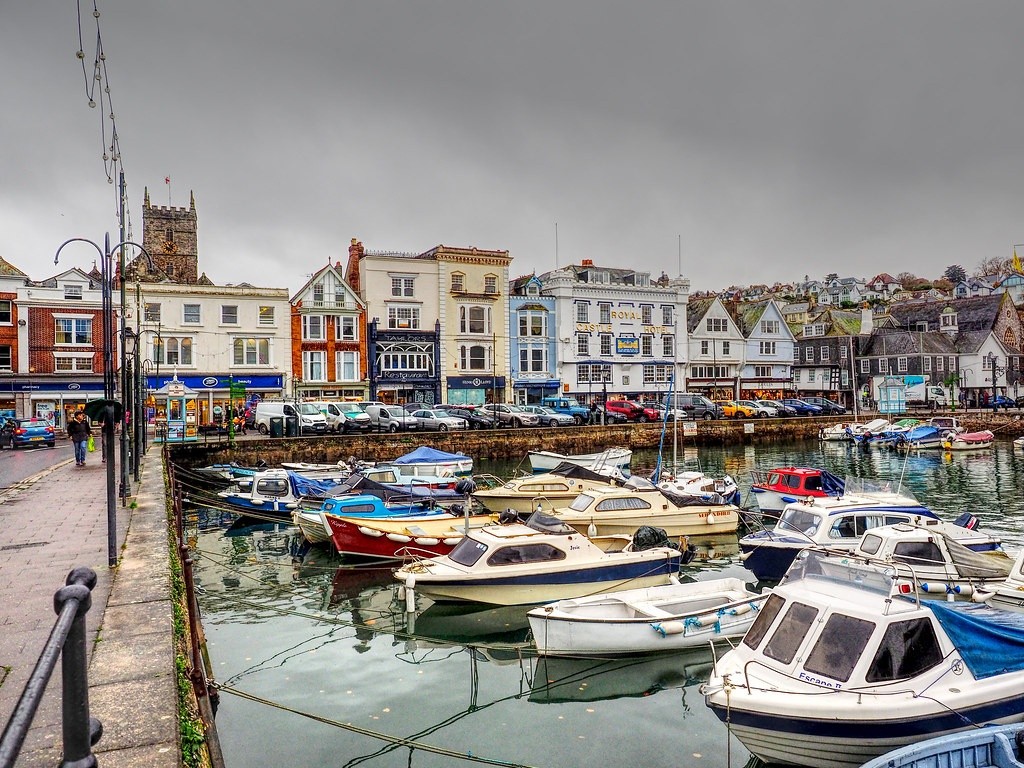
[286,417,299,437]
[270,417,283,438]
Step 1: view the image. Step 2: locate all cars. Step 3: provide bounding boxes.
[541,394,847,424]
[980,395,1016,408]
[518,405,577,427]
[1015,395,1024,407]
[400,403,541,431]
[0,417,54,450]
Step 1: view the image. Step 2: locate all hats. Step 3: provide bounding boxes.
[74,411,83,416]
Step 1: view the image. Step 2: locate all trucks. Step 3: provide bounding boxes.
[873,375,947,408]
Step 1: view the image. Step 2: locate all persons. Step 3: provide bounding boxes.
[586,399,598,426]
[98,410,121,463]
[67,411,92,466]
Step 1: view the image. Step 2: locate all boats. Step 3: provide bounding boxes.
[750,467,883,516]
[819,337,994,449]
[814,523,1024,613]
[524,578,775,653]
[698,522,1024,768]
[393,601,742,705]
[471,449,742,539]
[392,496,697,605]
[191,446,524,559]
[733,476,1001,581]
[858,722,1024,768]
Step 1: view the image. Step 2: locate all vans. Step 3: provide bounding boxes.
[255,402,419,435]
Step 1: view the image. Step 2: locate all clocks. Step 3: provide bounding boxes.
[163,241,177,254]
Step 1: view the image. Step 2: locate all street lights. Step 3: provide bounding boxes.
[600,369,610,425]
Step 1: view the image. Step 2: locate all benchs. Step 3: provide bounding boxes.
[628,600,673,619]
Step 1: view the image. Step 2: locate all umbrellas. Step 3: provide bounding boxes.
[82,398,123,422]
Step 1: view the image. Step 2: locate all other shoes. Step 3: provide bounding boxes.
[81,461,86,465]
[102,457,106,461]
[88,435,94,452]
[76,463,80,466]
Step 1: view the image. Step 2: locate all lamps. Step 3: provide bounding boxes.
[18,318,26,325]
